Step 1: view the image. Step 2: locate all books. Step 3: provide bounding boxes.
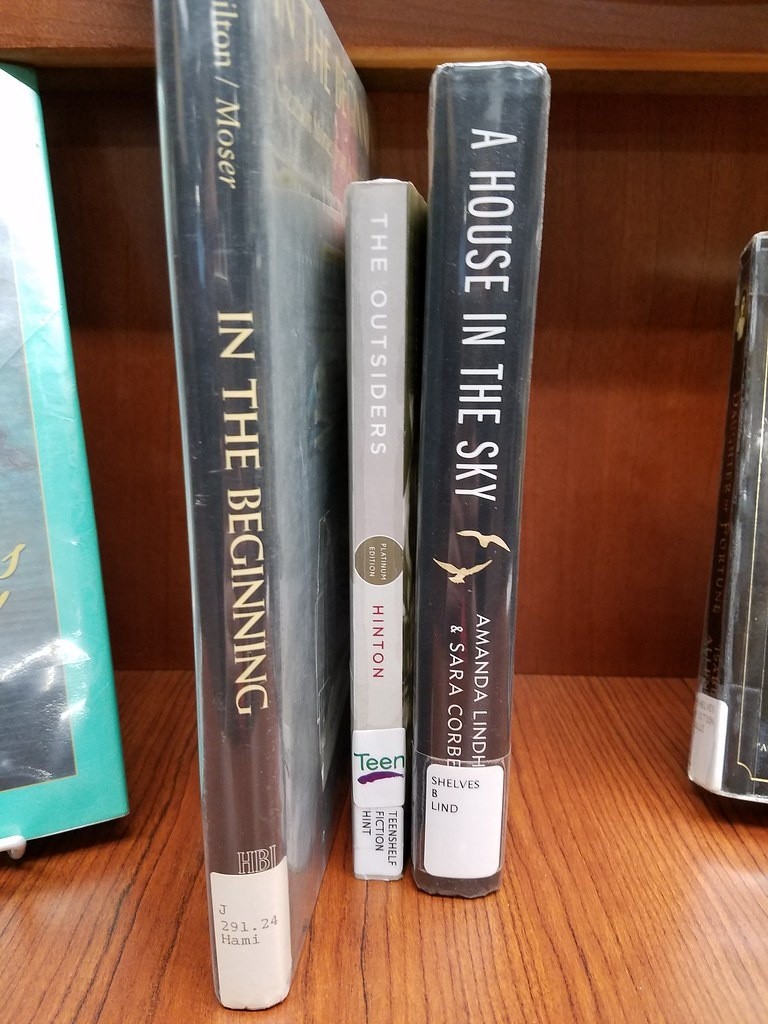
[680,229,768,806]
[149,0,556,1013]
[0,65,134,858]
